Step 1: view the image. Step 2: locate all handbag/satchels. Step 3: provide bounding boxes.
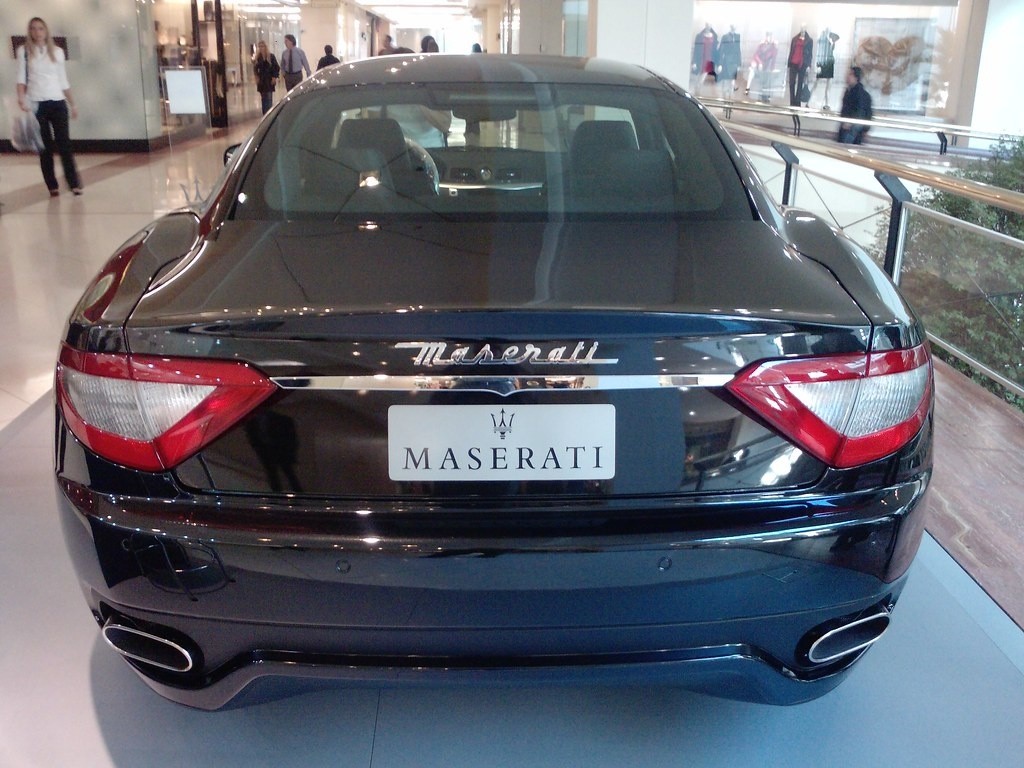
[268,53,280,80]
[10,95,45,152]
[800,72,810,102]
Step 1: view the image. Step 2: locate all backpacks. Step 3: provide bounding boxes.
[849,87,871,132]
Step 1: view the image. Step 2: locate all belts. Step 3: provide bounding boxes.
[285,71,301,75]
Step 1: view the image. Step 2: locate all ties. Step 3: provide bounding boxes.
[288,50,293,74]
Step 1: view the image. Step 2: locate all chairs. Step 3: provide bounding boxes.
[540,120,641,199]
[335,115,419,200]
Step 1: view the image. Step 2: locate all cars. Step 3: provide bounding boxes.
[51,53,938,716]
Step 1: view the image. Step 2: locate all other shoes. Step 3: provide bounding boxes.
[71,187,82,194]
[49,189,59,197]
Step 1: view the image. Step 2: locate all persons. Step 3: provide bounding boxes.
[355,35,450,148]
[253,41,279,116]
[691,23,741,109]
[281,35,311,92]
[787,23,839,109]
[836,67,873,143]
[463,43,483,137]
[15,17,85,197]
[316,45,341,72]
[744,32,777,93]
